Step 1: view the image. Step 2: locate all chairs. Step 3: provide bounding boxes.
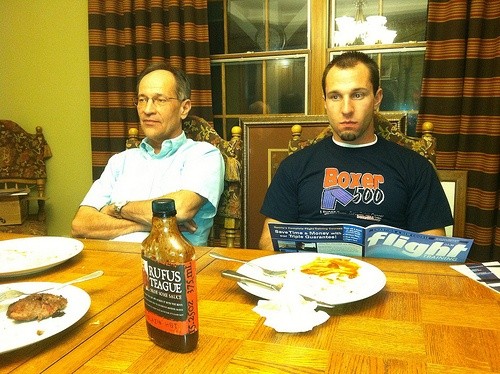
[0,119,54,237]
[288,111,438,185]
[127,114,245,248]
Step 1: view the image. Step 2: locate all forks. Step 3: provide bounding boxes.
[0,271,104,304]
[208,251,286,276]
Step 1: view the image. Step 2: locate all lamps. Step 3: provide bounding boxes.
[331,0,398,47]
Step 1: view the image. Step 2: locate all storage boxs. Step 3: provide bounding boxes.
[0,189,30,226]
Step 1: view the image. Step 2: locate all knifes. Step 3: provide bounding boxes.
[221,269,334,309]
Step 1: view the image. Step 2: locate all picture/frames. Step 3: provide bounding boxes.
[238,112,408,251]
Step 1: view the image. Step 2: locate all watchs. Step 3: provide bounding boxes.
[114,200,128,219]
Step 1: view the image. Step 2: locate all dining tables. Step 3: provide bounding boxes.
[0,231,500,374]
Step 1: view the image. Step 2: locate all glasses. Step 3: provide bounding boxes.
[134,95,178,106]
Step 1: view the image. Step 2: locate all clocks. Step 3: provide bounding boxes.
[255,24,288,52]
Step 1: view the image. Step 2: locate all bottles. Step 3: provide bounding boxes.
[140,199,200,354]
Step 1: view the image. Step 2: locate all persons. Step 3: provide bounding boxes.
[71,61,225,248]
[258,52,454,252]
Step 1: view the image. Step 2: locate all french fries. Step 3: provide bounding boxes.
[301,256,360,278]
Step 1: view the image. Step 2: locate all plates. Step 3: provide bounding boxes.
[0,282,91,353]
[0,237,84,279]
[237,253,387,306]
